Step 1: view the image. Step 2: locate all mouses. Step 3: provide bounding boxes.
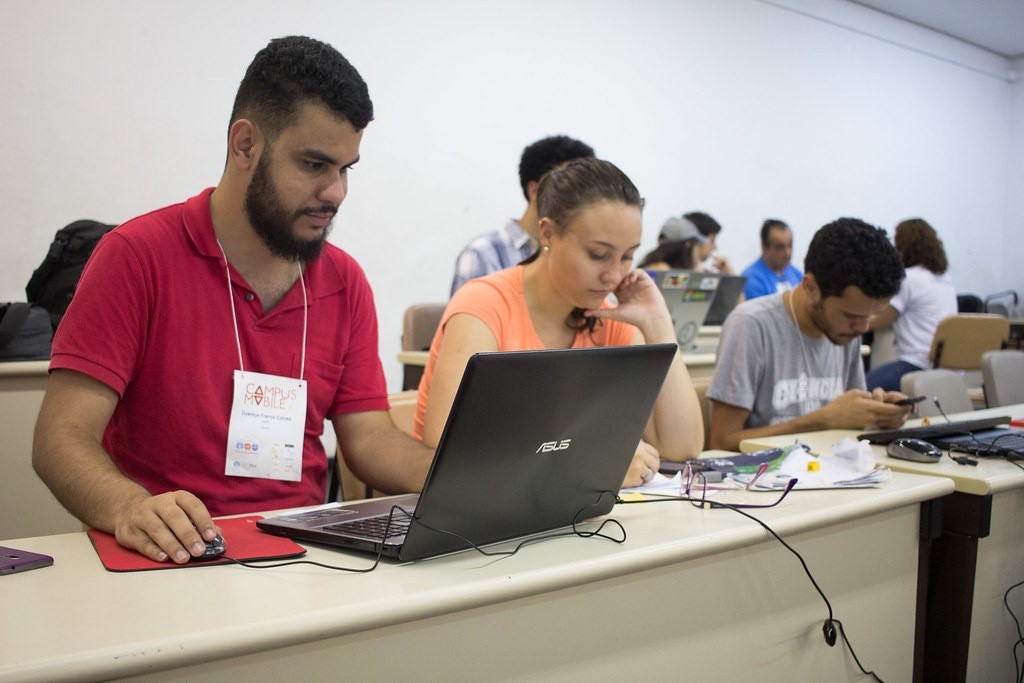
[886,438,942,463]
[167,521,226,561]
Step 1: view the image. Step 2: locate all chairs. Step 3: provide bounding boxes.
[981,350,1024,411]
[336,387,418,502]
[899,367,974,419]
[399,302,449,392]
[928,313,1010,371]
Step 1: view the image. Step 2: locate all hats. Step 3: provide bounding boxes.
[660,217,709,245]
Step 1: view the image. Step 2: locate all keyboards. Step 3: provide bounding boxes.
[856,416,1012,446]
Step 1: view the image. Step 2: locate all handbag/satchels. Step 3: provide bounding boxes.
[0,302,52,361]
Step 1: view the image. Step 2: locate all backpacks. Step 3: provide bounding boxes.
[26,220,118,332]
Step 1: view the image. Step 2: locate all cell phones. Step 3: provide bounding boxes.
[0,546,53,575]
[895,395,927,405]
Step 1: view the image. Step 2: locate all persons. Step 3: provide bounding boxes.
[413,157,705,489]
[739,219,803,301]
[638,211,746,304]
[706,217,913,452]
[865,219,958,393]
[31,35,435,564]
[449,135,595,299]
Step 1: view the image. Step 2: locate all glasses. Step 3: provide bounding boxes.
[679,461,798,509]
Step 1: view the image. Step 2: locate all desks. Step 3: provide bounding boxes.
[0,352,1024,683]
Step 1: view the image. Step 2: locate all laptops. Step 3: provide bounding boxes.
[934,428,1024,459]
[256,343,678,561]
[645,271,746,345]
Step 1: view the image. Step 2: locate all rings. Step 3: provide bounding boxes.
[643,469,652,481]
